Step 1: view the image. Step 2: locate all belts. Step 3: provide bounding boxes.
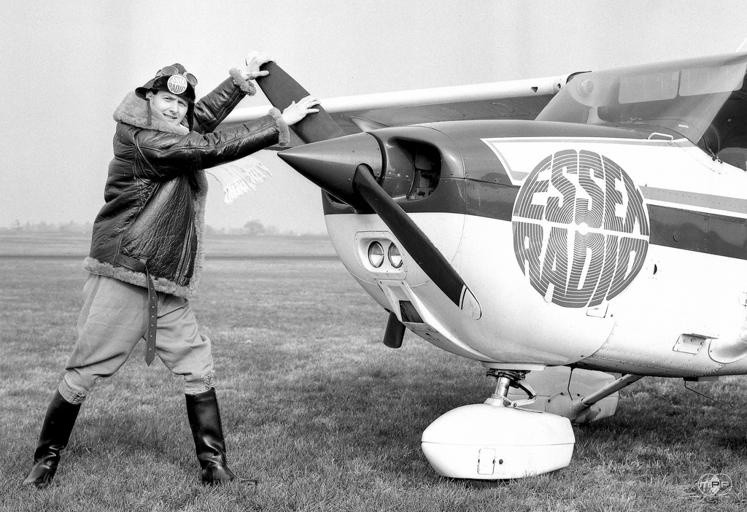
[117,253,157,366]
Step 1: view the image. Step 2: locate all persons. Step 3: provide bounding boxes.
[26,50,319,488]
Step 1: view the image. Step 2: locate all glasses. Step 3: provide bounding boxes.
[155,66,197,85]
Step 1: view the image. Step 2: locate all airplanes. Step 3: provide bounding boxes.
[213,48,747,488]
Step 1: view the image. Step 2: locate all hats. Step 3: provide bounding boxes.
[134,63,198,102]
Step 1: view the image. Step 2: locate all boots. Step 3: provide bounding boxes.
[22,387,82,490]
[185,387,257,487]
